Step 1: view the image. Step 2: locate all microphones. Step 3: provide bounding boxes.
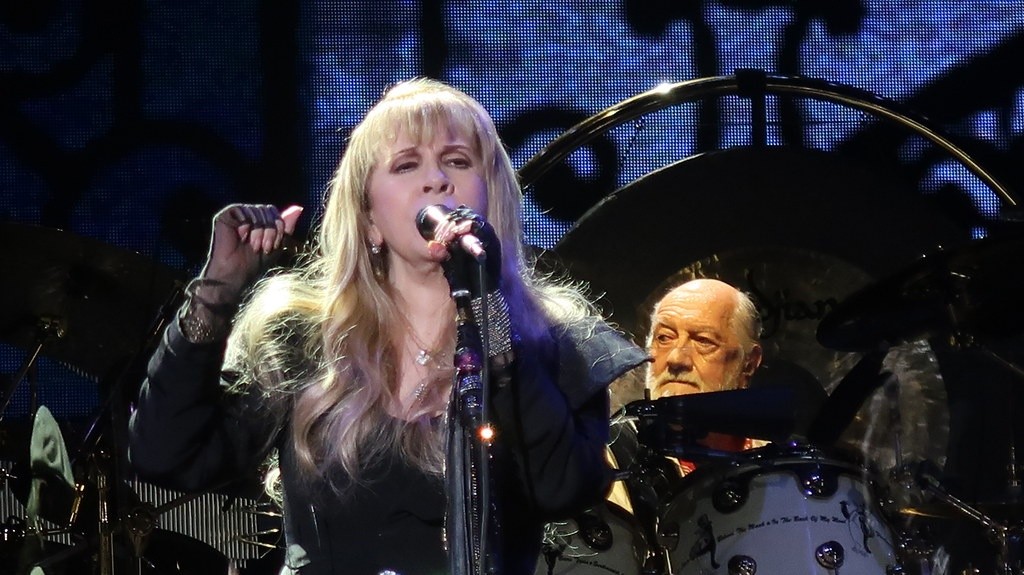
[416,204,486,261]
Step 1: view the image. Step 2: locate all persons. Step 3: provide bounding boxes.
[606,276,764,516]
[127,74,612,575]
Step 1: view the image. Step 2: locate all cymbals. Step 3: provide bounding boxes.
[0,219,183,369]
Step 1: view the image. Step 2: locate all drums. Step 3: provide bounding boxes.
[530,496,644,575]
[647,431,911,575]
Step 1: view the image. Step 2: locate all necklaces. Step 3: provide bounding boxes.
[399,294,447,400]
